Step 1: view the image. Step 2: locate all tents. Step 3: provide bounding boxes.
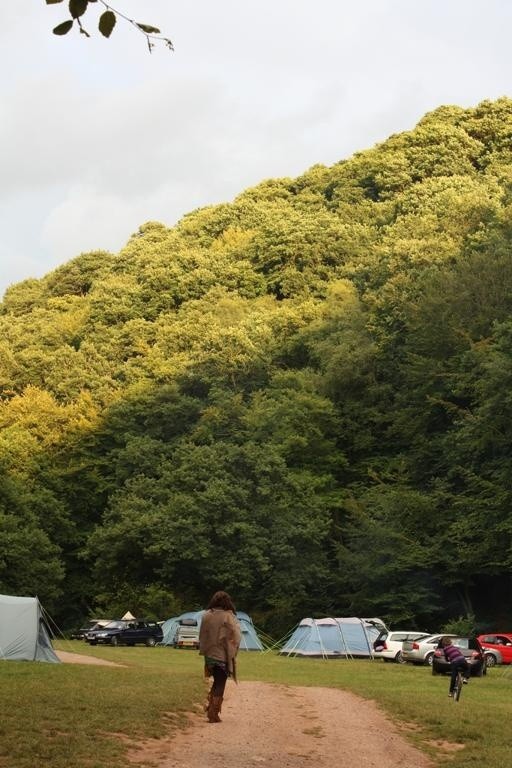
[155,610,283,654]
[260,617,392,661]
[119,611,139,628]
[0,594,76,664]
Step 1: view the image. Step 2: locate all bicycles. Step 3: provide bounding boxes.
[452,672,463,701]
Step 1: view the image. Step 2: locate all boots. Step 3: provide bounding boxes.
[205,693,223,722]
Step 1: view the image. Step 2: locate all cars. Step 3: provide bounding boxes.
[366,617,512,677]
[71,618,164,646]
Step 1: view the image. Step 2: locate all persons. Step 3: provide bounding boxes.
[443,637,471,697]
[199,592,241,722]
